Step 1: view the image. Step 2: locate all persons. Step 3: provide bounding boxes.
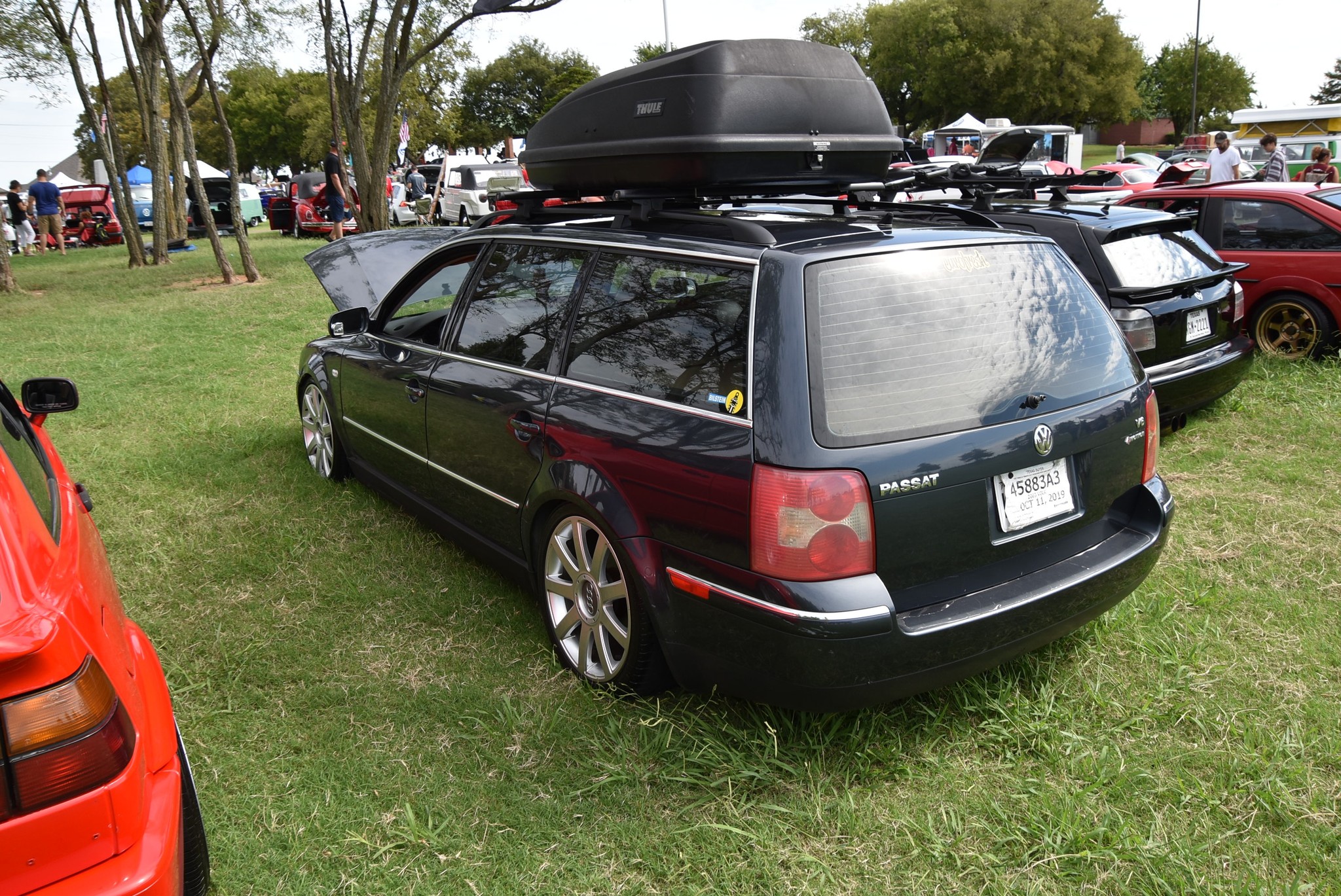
[0,167,93,257]
[949,137,958,155]
[1116,139,1126,163]
[386,177,394,230]
[1259,132,1290,182]
[1299,145,1339,183]
[255,175,291,191]
[961,140,976,154]
[323,139,352,242]
[407,165,431,226]
[1205,132,1241,182]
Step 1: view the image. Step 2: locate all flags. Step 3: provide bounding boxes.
[101,108,108,134]
[398,113,410,165]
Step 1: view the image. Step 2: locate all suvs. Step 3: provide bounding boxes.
[296,39,1174,693]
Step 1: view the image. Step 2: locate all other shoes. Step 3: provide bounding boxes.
[24,251,36,257]
[326,236,335,243]
[419,224,424,227]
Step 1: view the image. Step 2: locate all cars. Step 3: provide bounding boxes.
[0,177,287,252]
[268,154,614,238]
[717,126,1341,438]
[1,379,209,895]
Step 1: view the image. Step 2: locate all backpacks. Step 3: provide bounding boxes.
[94,223,110,242]
[1305,165,1333,183]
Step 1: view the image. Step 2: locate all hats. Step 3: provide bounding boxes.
[10,180,21,187]
[1214,131,1228,144]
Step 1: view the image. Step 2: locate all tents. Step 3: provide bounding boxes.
[47,171,88,189]
[922,113,990,157]
[118,157,304,186]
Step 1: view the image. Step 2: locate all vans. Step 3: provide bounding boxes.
[1234,137,1341,179]
